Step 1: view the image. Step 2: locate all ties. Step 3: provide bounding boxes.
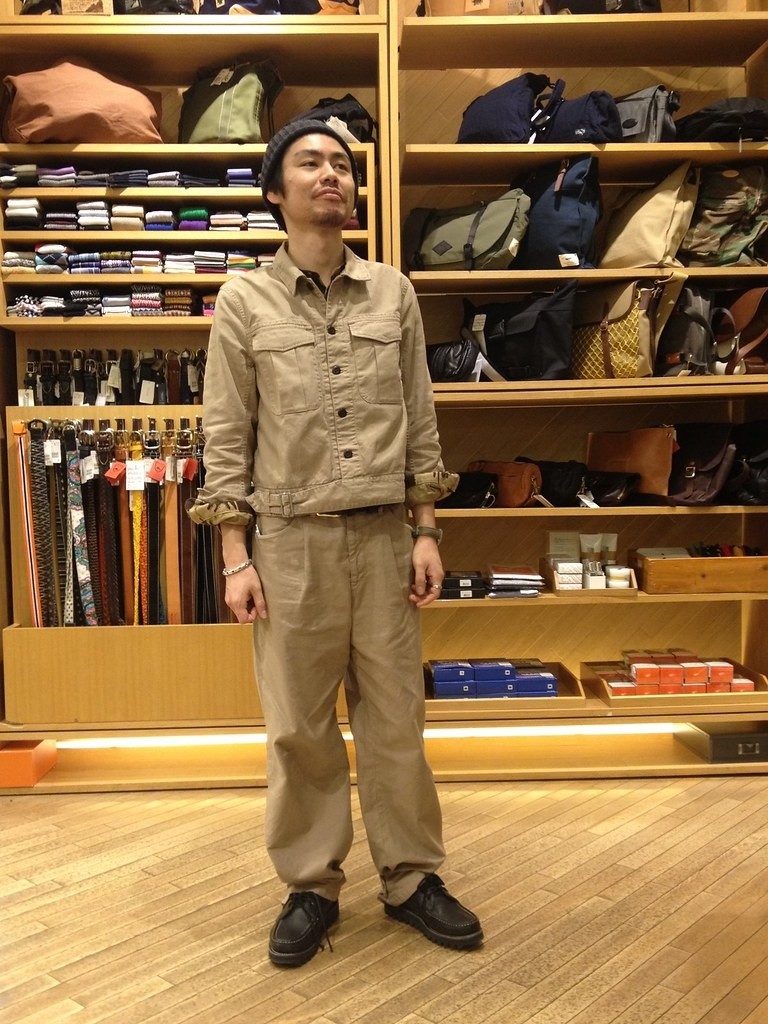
[0,154,365,318]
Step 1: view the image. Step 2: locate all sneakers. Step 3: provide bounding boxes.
[383,869,484,951]
[268,891,339,966]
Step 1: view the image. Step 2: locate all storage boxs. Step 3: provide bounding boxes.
[423,647,755,699]
[626,548,768,595]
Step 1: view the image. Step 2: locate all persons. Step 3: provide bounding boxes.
[191,119,484,965]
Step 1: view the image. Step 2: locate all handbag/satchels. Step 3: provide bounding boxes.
[467,460,542,509]
[434,471,498,508]
[514,456,590,506]
[463,272,768,382]
[457,70,768,144]
[288,93,380,164]
[3,55,162,145]
[425,339,476,382]
[588,469,641,506]
[178,54,286,145]
[403,156,768,270]
[18,0,662,15]
[585,423,768,505]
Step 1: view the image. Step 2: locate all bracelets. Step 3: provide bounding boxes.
[223,559,253,576]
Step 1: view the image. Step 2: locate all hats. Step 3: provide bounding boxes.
[260,119,360,210]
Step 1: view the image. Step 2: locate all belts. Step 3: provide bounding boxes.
[11,348,257,626]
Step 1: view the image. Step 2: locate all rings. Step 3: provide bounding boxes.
[430,584,442,590]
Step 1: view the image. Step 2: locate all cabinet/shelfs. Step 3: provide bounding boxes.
[390,0,768,723]
[0,24,392,733]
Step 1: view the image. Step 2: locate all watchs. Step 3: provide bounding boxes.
[412,526,443,546]
